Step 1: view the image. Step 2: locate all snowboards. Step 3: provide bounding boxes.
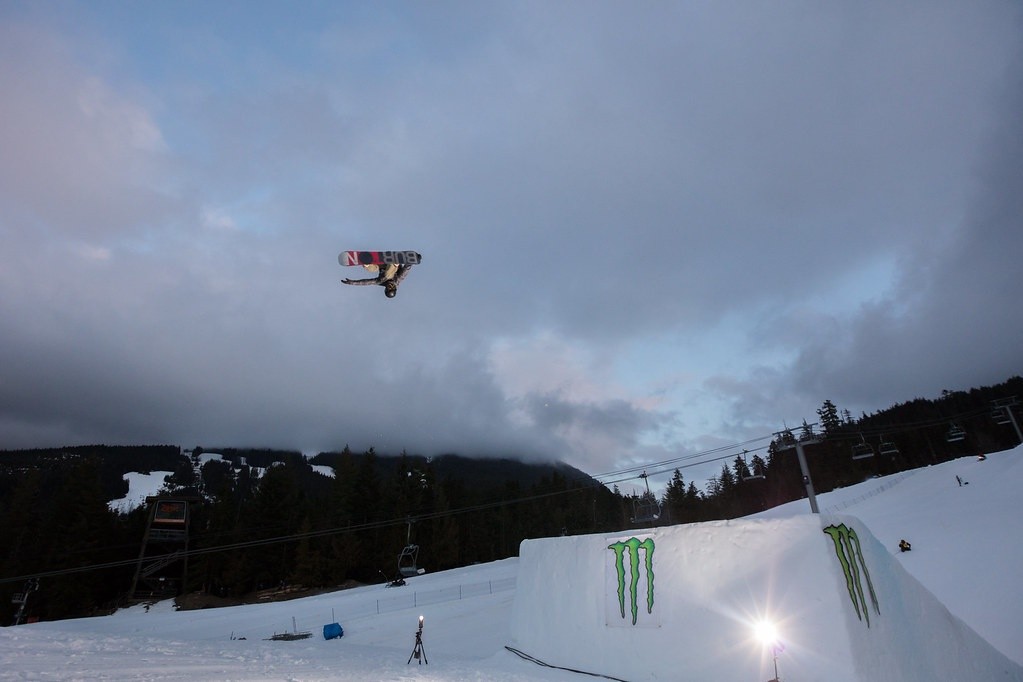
[338,250,421,267]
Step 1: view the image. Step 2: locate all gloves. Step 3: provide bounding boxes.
[341,278,350,284]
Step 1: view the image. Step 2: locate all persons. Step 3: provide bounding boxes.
[898,540,911,553]
[341,263,412,298]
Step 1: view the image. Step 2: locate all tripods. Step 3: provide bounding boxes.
[408,630,428,665]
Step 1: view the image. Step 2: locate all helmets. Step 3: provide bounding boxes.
[383,289,397,298]
